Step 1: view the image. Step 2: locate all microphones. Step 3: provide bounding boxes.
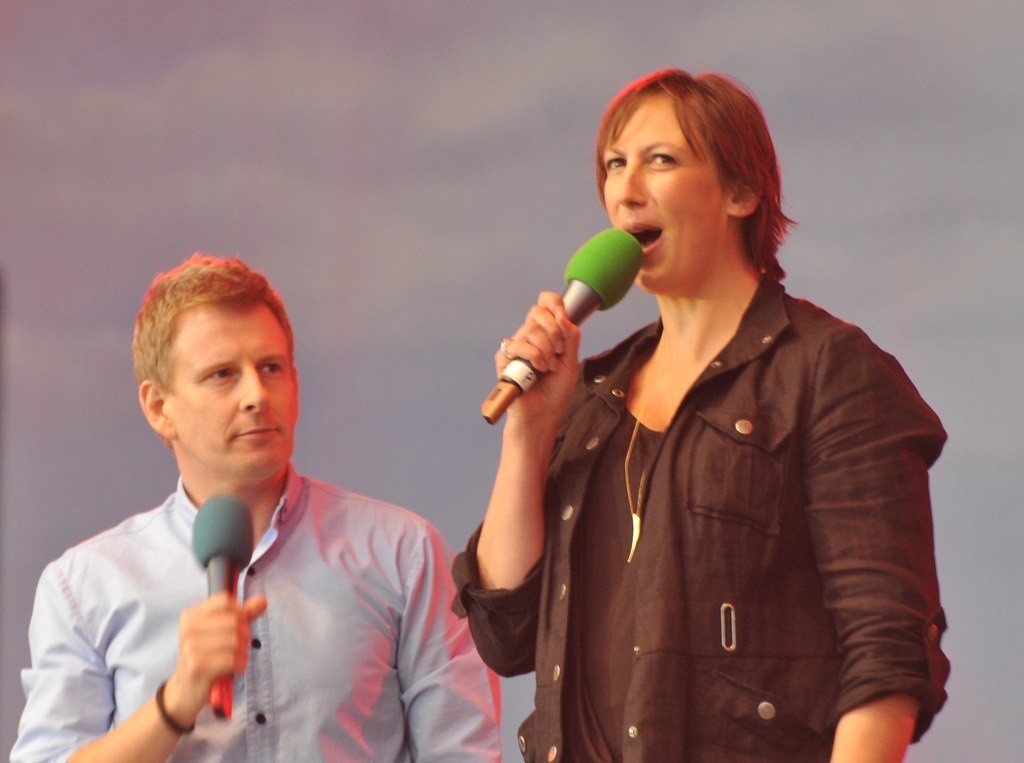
[478,230,642,423]
[192,494,253,718]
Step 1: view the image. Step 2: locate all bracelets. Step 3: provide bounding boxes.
[153,684,197,733]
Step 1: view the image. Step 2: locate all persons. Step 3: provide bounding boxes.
[3,250,511,763]
[451,64,960,763]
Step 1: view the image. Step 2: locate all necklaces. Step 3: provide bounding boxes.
[620,340,682,569]
